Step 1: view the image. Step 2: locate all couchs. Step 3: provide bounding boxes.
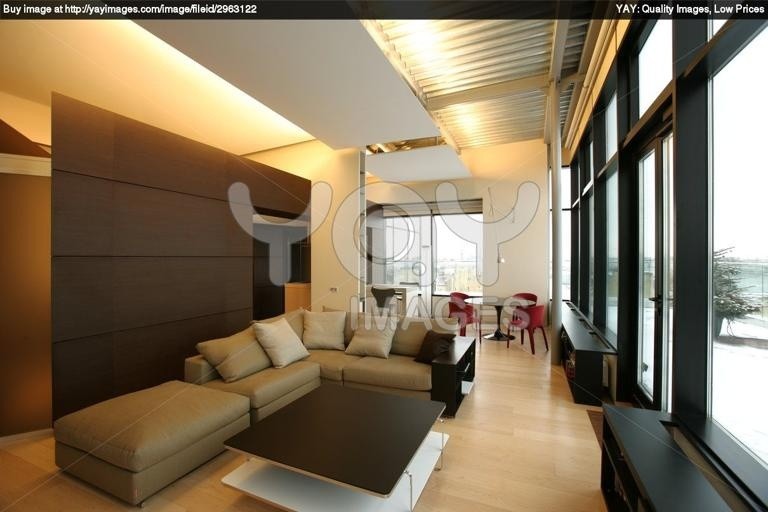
[185,308,321,427]
[250,307,476,418]
[53,379,250,507]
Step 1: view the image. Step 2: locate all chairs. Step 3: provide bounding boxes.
[511,292,537,331]
[371,287,395,314]
[507,305,549,354]
[449,292,481,343]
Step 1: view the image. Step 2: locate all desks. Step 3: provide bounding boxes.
[464,296,537,340]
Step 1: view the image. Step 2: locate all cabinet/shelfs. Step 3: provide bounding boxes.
[550,299,617,406]
[601,400,735,512]
[365,283,418,317]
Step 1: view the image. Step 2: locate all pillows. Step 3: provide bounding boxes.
[345,315,400,358]
[252,315,311,370]
[412,330,456,363]
[195,320,270,383]
[303,307,346,351]
